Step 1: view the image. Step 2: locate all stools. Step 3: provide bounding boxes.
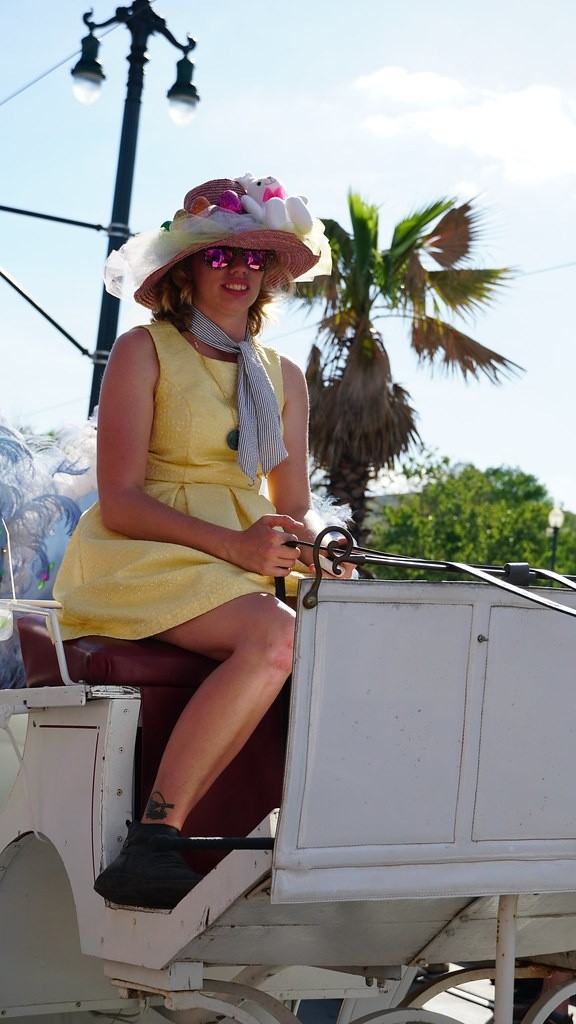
[6,596,300,877]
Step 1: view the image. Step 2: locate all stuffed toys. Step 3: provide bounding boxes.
[236,171,312,234]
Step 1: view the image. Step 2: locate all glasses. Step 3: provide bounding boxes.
[203,246,276,272]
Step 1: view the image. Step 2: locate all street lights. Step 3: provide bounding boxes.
[73,0,201,420]
[548,498,563,587]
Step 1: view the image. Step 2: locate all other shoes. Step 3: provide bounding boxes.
[93,819,205,909]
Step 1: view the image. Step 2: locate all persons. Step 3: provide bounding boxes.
[53,180,357,907]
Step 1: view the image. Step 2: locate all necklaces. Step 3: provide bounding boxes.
[190,330,239,450]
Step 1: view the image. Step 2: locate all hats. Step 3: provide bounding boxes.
[104,172,332,310]
